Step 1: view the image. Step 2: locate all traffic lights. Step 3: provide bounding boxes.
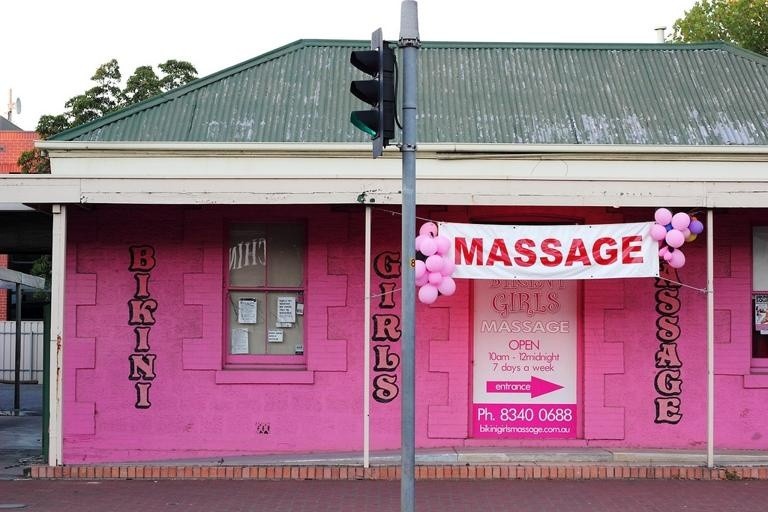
[350,38,398,152]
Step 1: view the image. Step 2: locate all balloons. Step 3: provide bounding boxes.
[435,234,452,257]
[415,256,458,305]
[419,239,438,257]
[419,222,438,238]
[415,236,426,252]
[649,207,703,269]
